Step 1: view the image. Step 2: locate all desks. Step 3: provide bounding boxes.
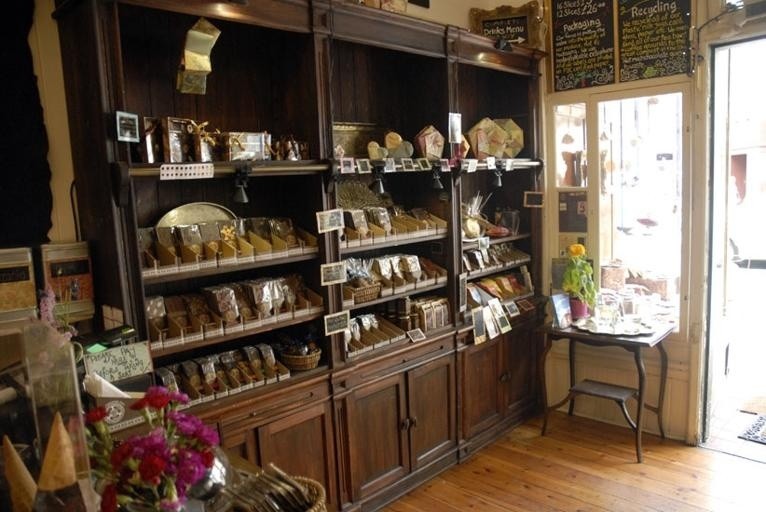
[532,318,676,463]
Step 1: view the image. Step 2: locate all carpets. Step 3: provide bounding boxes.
[738,416,766,444]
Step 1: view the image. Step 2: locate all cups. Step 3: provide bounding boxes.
[593,288,642,333]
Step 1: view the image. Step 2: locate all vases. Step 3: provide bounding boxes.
[570,298,588,319]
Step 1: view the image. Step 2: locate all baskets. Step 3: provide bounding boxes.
[343,282,380,303]
[205,476,327,512]
[281,348,322,371]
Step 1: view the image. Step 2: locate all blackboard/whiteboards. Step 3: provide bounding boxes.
[480,14,531,45]
[552,0,614,91]
[618,0,691,81]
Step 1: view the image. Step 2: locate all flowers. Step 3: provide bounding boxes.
[564,243,598,306]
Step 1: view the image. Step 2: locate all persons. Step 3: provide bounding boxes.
[122,116,136,138]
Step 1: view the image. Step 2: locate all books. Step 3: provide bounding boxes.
[548,290,573,329]
